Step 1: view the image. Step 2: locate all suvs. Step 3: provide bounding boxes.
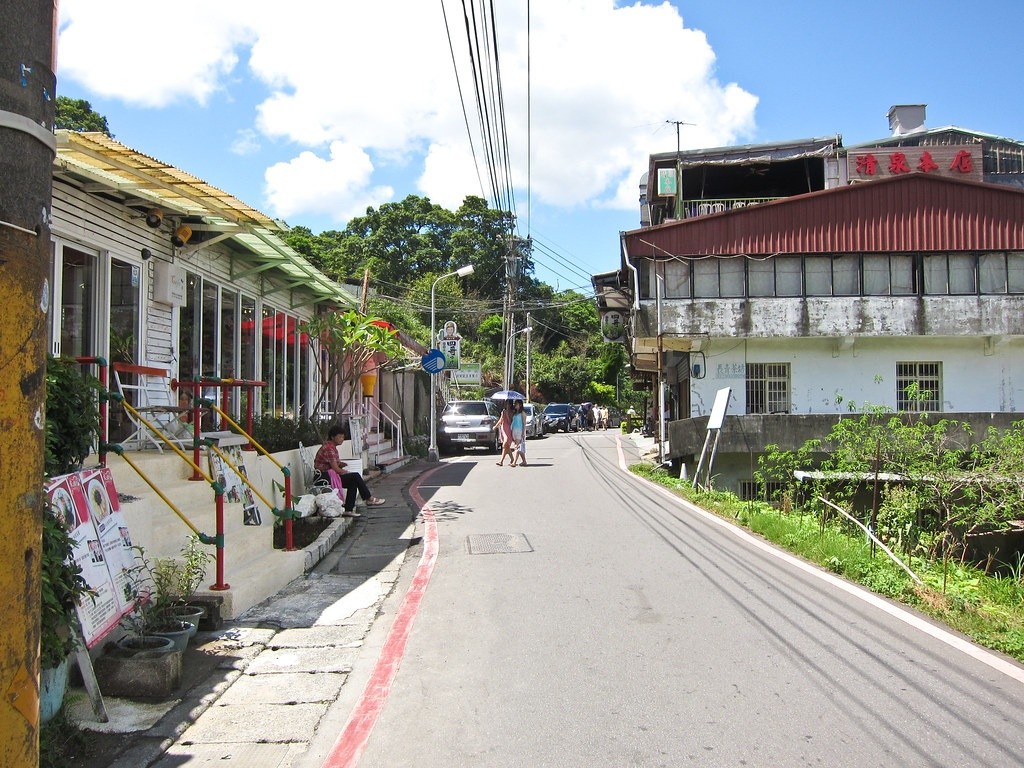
[436,399,501,457]
[542,401,581,434]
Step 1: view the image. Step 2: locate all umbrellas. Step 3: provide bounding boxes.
[491,391,526,409]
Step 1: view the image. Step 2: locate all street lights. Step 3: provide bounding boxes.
[504,327,533,391]
[427,264,475,463]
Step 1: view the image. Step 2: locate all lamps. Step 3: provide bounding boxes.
[131,207,163,228]
[161,225,192,247]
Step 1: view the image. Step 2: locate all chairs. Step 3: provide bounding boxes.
[299,441,344,506]
[113,362,207,453]
[699,203,712,216]
[712,203,725,213]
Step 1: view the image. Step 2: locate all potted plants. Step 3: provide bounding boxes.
[40,419,100,725]
[155,534,217,634]
[117,565,175,652]
[140,556,195,655]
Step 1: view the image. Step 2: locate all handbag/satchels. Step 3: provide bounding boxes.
[517,435,526,454]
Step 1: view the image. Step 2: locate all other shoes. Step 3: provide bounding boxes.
[342,510,361,518]
[508,458,514,465]
[496,462,503,466]
[519,461,527,466]
[510,463,516,467]
[366,497,386,506]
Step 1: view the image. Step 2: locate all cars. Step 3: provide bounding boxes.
[605,405,622,428]
[514,402,545,439]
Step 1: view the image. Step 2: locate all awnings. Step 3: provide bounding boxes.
[241,313,317,349]
[370,319,430,361]
[55,129,364,311]
[591,270,633,316]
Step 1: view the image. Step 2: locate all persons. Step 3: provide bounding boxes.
[443,321,455,340]
[314,426,385,517]
[492,399,528,467]
[571,404,636,432]
